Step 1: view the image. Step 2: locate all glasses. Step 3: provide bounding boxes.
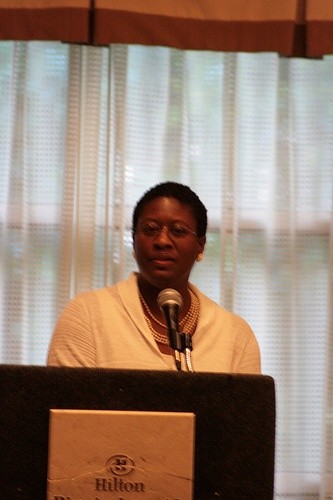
[135,221,198,239]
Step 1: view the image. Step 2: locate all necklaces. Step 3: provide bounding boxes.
[138,286,200,346]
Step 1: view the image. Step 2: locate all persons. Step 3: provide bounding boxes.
[46,181,261,377]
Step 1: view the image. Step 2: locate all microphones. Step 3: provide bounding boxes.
[155,288,184,372]
